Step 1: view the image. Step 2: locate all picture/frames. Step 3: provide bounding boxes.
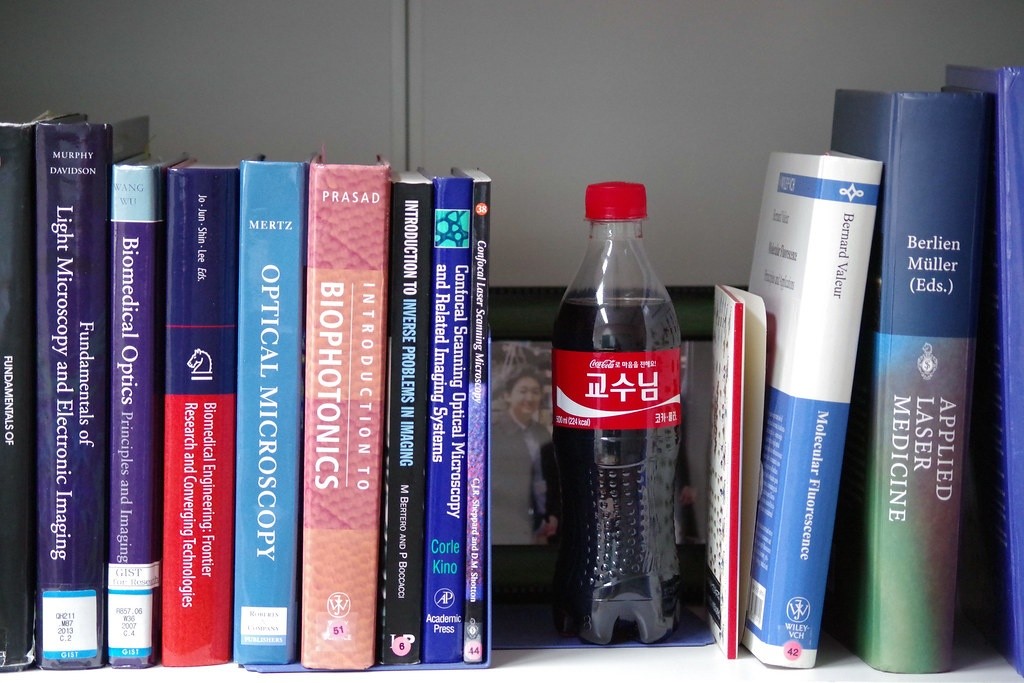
[488,286,749,604]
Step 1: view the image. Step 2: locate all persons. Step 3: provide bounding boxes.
[491,365,557,549]
[672,389,702,544]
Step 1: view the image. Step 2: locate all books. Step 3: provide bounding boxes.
[0,112,492,676]
[708,60,1022,676]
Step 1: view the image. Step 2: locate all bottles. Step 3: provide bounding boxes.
[545,182,684,644]
